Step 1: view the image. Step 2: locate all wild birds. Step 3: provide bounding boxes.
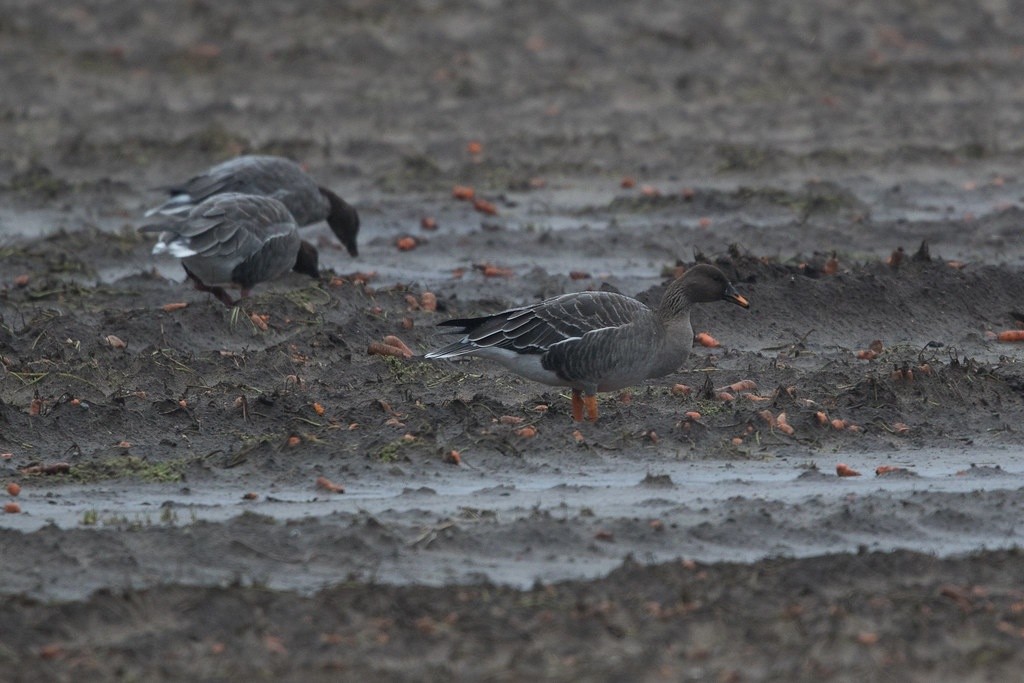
[136,194,322,316]
[169,155,361,257]
[422,264,751,422]
